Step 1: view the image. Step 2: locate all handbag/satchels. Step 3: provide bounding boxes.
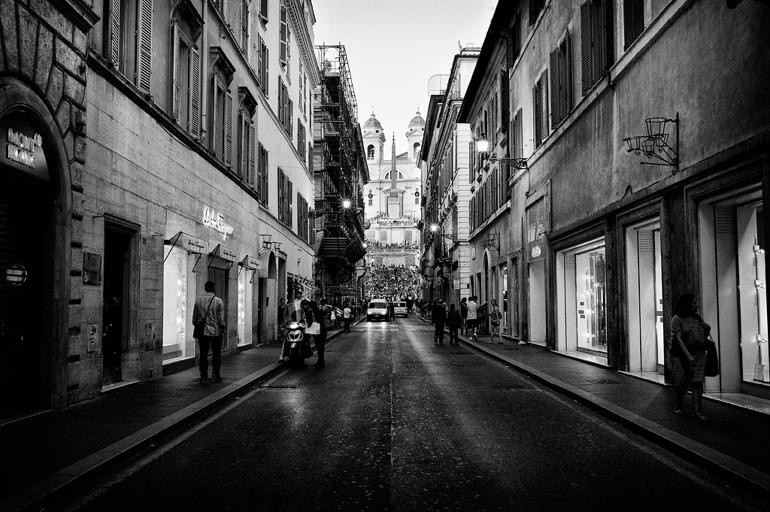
[193,320,206,338]
[304,322,321,335]
[705,333,719,379]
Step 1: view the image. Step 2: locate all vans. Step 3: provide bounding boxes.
[367,298,391,321]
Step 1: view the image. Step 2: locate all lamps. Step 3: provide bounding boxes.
[430,224,456,243]
[309,198,353,219]
[475,131,530,170]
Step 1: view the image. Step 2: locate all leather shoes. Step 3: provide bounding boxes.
[201,373,223,385]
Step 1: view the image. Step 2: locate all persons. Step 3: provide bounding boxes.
[192,281,225,384]
[669,293,711,420]
[415,296,479,346]
[487,299,503,344]
[388,302,396,321]
[277,293,367,368]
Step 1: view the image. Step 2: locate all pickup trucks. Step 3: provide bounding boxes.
[393,301,409,318]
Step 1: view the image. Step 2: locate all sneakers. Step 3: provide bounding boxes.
[469,333,478,341]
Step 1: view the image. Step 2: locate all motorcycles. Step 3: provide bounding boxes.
[282,321,311,371]
